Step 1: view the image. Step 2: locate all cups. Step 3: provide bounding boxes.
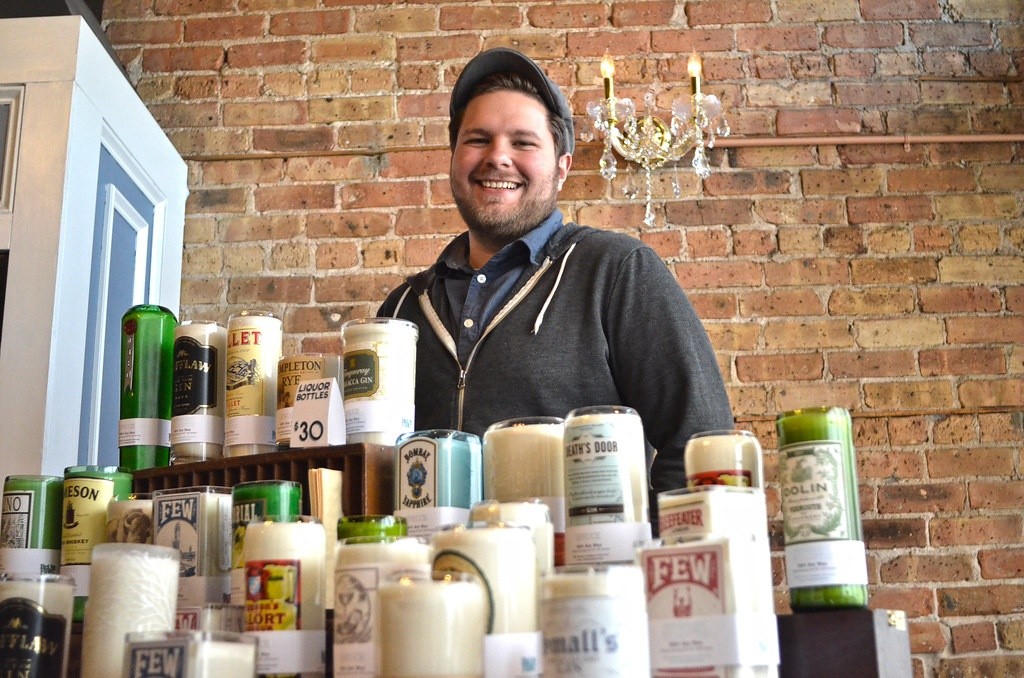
[0,305,866,678]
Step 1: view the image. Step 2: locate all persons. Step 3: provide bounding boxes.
[376,49,732,539]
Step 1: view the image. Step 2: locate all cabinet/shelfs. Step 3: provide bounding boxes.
[135,440,394,525]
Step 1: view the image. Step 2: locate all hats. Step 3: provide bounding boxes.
[449,46,575,157]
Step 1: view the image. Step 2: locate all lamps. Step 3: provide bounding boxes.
[581,45,731,226]
